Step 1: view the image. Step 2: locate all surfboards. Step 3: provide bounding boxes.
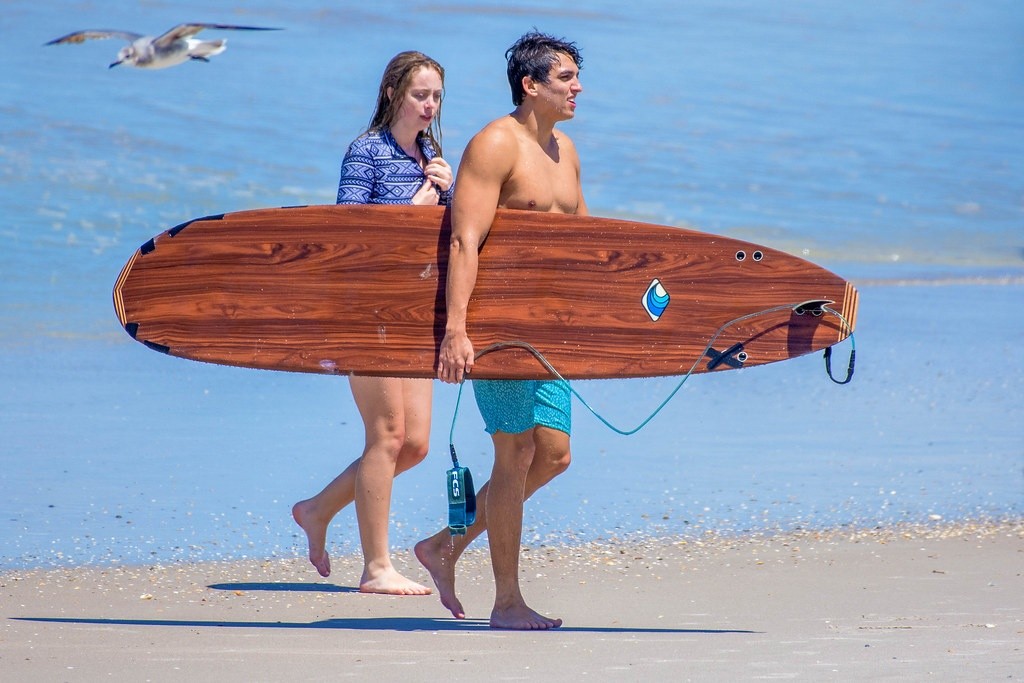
[106,200,864,383]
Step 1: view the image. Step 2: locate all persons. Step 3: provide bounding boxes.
[292,50,454,596]
[413,32,590,630]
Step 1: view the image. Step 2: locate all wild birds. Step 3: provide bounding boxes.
[39,19,287,70]
